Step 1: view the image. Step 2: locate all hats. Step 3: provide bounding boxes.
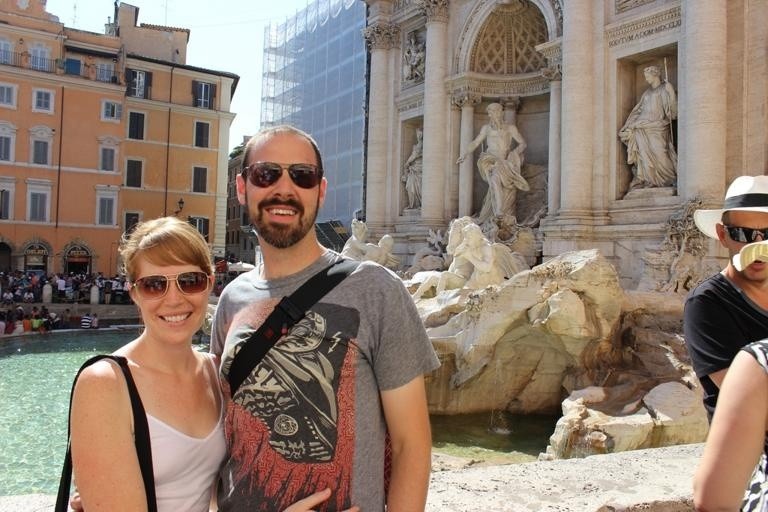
[731,239,767,272]
[692,174,768,240]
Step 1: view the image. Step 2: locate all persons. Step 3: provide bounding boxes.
[0,264,134,342]
[215,281,224,297]
[402,37,420,79]
[615,64,679,190]
[352,231,394,269]
[410,43,426,81]
[689,337,768,511]
[64,124,445,511]
[680,173,768,429]
[343,219,369,262]
[453,103,530,222]
[458,222,498,289]
[399,128,425,211]
[64,214,366,512]
[408,212,475,302]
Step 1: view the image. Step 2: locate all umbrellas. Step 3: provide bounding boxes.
[228,260,255,272]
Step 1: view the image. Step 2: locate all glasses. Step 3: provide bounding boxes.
[721,222,768,244]
[127,269,212,301]
[242,160,324,190]
